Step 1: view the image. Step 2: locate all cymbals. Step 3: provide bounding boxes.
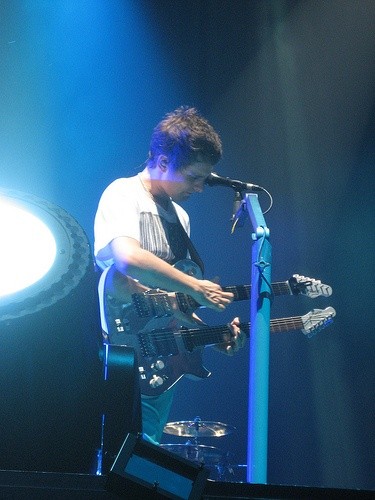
[159,443,229,463]
[161,421,237,437]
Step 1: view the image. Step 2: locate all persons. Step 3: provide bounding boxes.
[88,102,245,494]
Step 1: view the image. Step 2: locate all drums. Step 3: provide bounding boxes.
[203,464,227,482]
[226,465,247,483]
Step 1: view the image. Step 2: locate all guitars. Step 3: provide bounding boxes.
[97,261,333,334]
[108,306,337,395]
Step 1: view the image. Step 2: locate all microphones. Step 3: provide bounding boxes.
[205,173,264,194]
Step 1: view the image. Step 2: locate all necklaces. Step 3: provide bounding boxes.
[137,172,173,213]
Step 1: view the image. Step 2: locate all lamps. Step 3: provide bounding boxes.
[0,188,94,470]
